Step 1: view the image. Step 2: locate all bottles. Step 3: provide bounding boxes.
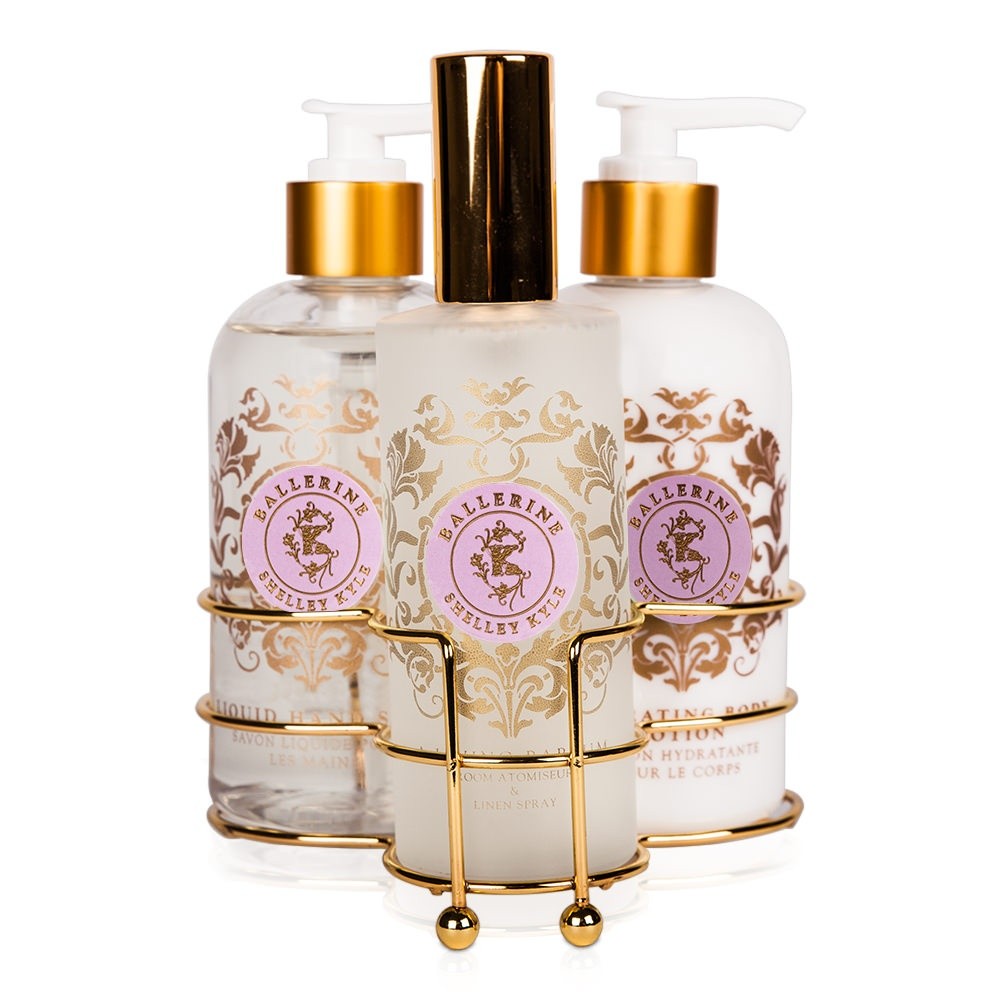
[384,48,636,885]
